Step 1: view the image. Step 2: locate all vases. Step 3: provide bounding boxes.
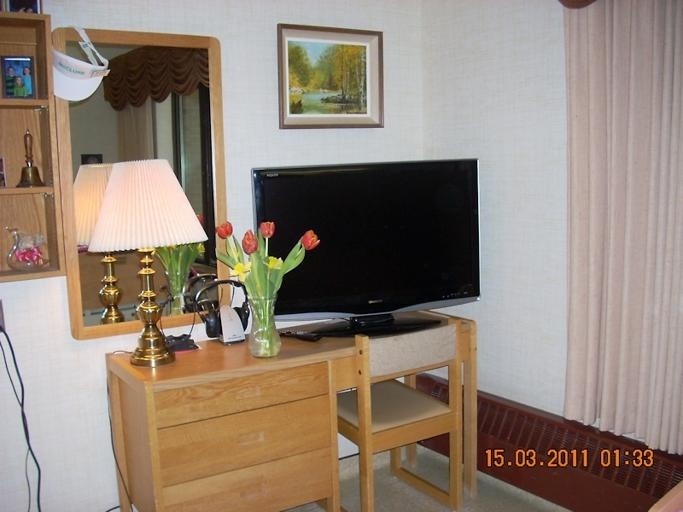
[247,299,282,358]
[165,271,190,317]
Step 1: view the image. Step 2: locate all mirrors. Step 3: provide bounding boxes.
[51,26,232,341]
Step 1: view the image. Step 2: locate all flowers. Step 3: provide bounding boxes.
[154,213,206,313]
[214,221,320,354]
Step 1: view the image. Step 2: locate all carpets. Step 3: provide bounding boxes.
[285,442,568,511]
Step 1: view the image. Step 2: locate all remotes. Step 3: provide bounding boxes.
[278,328,322,341]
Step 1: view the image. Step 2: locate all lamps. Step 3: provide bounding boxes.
[73,164,132,324]
[88,158,210,366]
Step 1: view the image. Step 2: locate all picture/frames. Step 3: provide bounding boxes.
[0,54,38,99]
[277,23,384,128]
[81,154,103,163]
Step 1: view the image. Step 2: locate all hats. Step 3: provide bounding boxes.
[51,25,110,102]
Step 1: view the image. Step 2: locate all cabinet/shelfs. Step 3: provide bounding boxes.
[1,12,67,283]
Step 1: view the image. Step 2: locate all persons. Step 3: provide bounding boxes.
[22,66,33,95]
[13,76,26,97]
[4,66,15,96]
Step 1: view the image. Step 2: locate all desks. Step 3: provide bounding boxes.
[107,313,480,512]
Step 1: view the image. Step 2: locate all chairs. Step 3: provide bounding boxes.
[317,320,464,511]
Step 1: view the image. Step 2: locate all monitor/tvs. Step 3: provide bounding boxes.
[250,158,481,339]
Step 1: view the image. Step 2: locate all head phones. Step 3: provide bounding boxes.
[193,279,251,338]
